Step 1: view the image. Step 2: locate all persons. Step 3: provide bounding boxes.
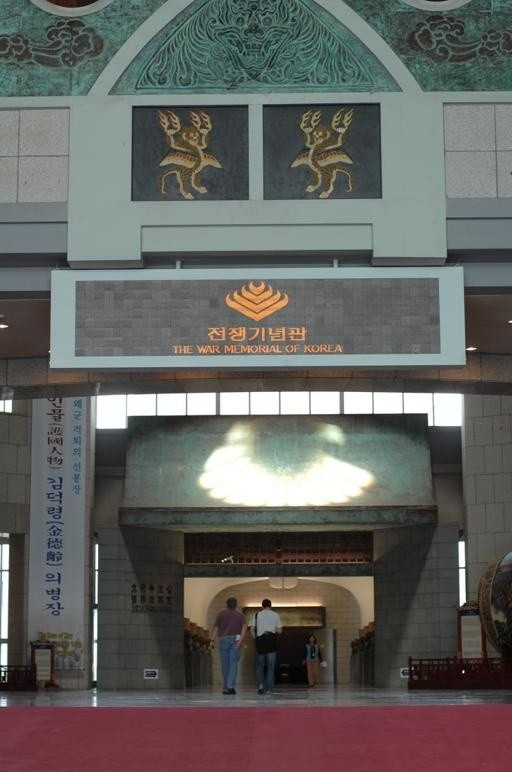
[250,599,283,694]
[303,634,323,687]
[207,598,248,694]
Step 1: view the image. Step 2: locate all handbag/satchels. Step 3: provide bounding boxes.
[254,632,280,654]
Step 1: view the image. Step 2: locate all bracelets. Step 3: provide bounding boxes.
[210,639,215,641]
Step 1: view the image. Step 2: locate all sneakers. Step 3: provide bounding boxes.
[257,688,265,694]
[223,687,237,694]
